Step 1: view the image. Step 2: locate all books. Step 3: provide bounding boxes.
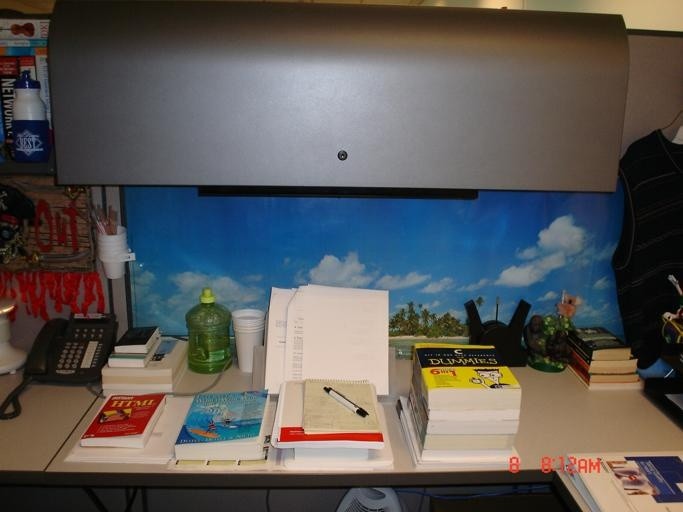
[564,451,683,512]
[0,13,54,162]
[571,326,646,392]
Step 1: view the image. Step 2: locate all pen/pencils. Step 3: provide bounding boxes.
[323,386,369,417]
[90,204,117,235]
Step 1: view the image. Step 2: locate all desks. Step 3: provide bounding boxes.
[0,356,682,510]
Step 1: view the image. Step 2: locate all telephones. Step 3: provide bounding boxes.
[23,317,114,383]
